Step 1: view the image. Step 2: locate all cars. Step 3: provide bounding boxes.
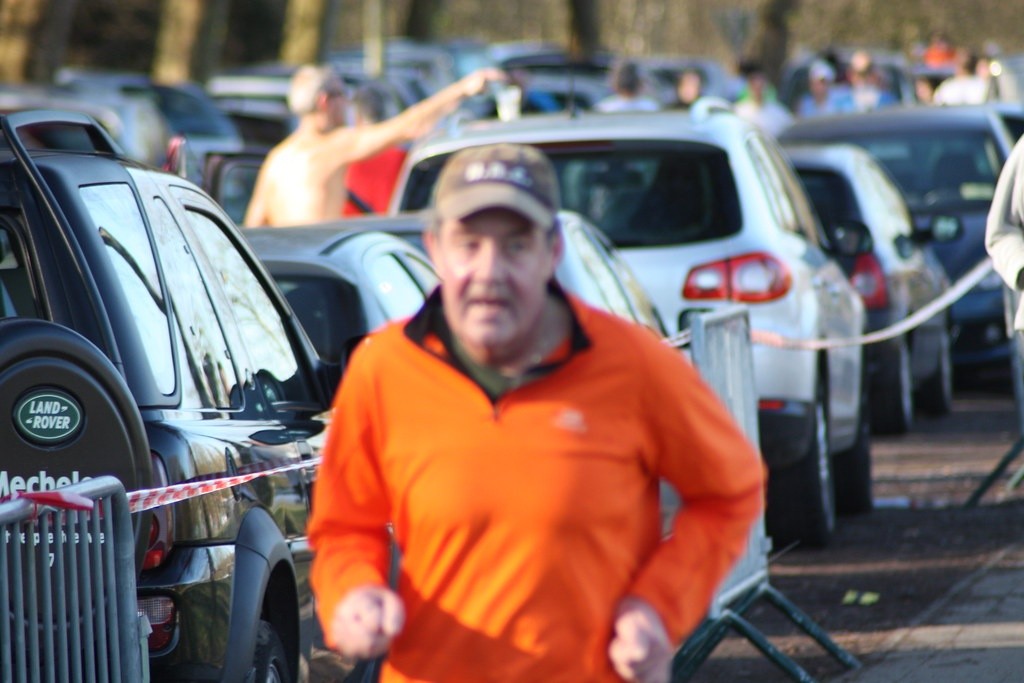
[778,104,1023,434]
[229,208,680,538]
[1,39,758,195]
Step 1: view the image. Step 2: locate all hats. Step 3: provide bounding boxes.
[431,143,557,228]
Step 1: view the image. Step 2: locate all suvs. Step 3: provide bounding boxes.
[388,94,875,544]
[0,108,369,683]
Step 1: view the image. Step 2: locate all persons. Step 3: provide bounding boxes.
[510,50,997,141]
[984,133,1024,341]
[243,62,511,228]
[309,143,768,683]
